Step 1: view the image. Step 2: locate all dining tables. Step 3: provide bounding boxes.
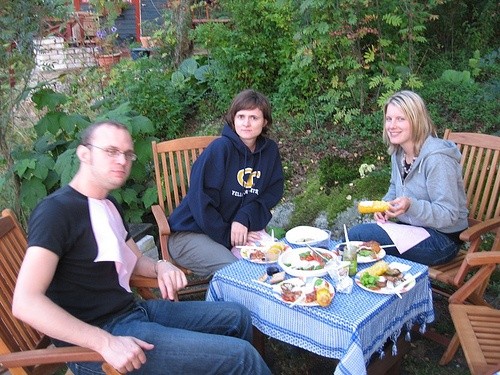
[205,238,435,375]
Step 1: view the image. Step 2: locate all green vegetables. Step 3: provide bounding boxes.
[360,272,378,287]
[284,250,330,270]
[358,249,373,256]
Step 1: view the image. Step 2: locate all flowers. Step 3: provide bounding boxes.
[97,27,117,55]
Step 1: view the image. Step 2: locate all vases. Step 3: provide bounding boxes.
[97,50,122,67]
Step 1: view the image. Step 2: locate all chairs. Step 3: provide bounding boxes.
[0,129,500,375]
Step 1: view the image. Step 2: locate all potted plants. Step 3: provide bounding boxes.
[140,20,157,47]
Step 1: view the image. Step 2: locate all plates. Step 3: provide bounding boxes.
[277,247,339,278]
[273,277,335,307]
[241,242,292,264]
[333,241,386,263]
[285,225,328,247]
[355,266,416,294]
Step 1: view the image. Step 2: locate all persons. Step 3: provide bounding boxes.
[11,120,272,375]
[168,89,285,276]
[343,90,469,267]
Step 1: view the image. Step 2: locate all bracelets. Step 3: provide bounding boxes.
[155,259,169,275]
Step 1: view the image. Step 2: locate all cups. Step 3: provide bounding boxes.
[342,246,358,275]
[317,230,332,250]
[264,248,282,275]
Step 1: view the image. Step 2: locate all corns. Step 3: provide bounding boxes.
[316,288,331,307]
[266,244,282,260]
[366,260,388,276]
[358,200,390,213]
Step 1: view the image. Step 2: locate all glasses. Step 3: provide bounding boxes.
[86,142,137,164]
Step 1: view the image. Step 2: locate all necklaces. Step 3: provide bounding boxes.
[401,152,417,174]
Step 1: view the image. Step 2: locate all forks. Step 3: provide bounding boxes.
[357,245,397,252]
[252,279,284,294]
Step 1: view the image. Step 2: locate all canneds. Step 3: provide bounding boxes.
[343,245,358,275]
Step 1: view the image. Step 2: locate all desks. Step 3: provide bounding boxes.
[41,14,85,47]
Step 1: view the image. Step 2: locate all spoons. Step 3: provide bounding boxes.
[289,285,313,308]
[387,280,402,300]
